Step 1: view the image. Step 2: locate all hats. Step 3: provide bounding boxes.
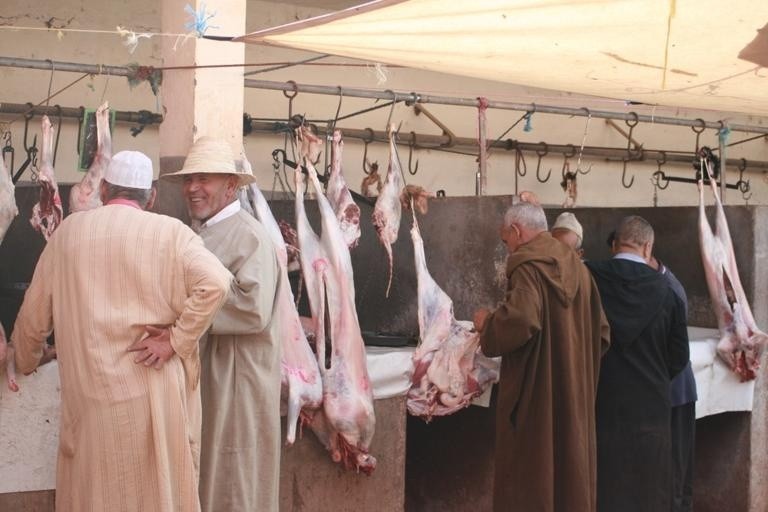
[550,211,584,244]
[159,136,256,187]
[103,149,154,191]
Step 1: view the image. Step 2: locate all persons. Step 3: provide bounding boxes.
[161,135,282,512]
[470,203,611,511]
[605,226,697,511]
[7,149,232,511]
[549,204,597,282]
[587,213,690,512]
